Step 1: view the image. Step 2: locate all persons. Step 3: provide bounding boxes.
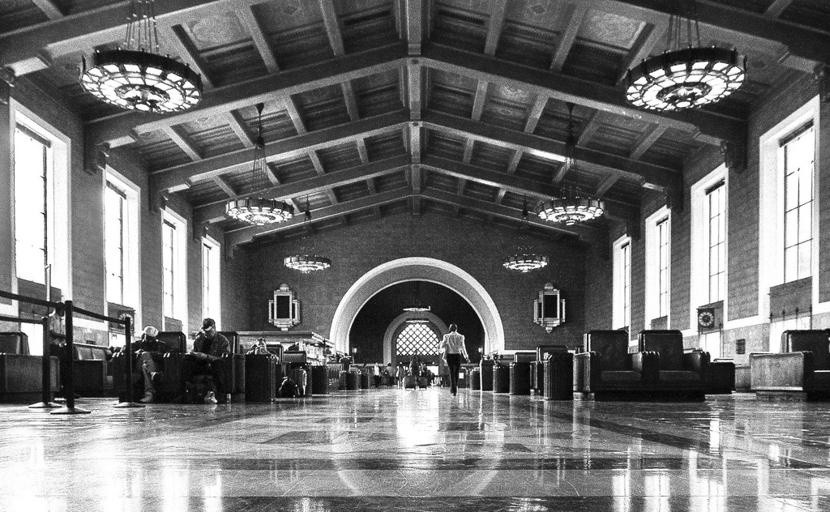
[440,323,471,396]
[48,301,81,399]
[338,349,435,387]
[246,337,279,364]
[132,326,168,403]
[190,318,230,403]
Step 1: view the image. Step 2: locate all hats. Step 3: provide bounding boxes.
[142,325,159,338]
[200,318,216,332]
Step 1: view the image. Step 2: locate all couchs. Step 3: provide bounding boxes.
[749,328,830,401]
[478,344,573,401]
[573,330,736,401]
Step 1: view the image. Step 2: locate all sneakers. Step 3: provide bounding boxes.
[152,372,164,381]
[450,385,458,397]
[204,390,218,404]
[139,394,157,403]
[226,393,232,404]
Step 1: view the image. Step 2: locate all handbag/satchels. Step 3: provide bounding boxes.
[383,369,390,378]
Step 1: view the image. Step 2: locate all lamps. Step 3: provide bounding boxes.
[224,102,329,274]
[622,1,750,114]
[77,1,204,115]
[504,102,605,273]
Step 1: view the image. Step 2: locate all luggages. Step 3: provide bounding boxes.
[418,372,428,390]
[404,370,416,390]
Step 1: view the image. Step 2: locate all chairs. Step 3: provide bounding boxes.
[114,332,326,407]
